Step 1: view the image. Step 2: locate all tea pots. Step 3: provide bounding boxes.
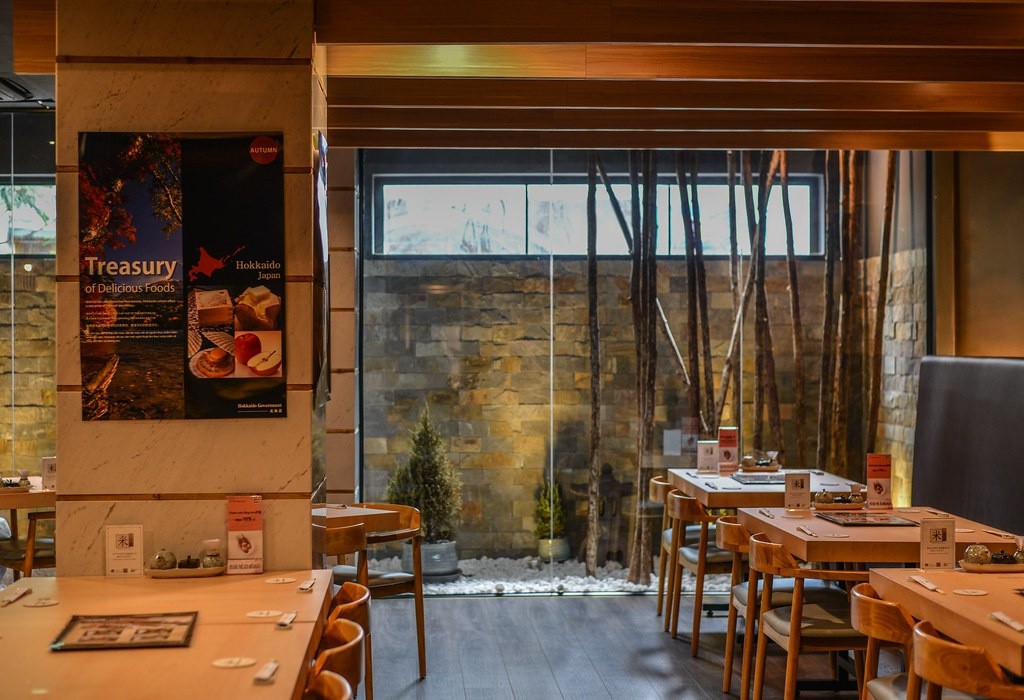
[742,455,755,467]
[964,541,992,564]
[150,548,176,569]
[814,489,833,503]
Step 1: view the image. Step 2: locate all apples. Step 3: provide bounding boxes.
[235,333,281,374]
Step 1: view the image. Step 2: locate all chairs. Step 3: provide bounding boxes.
[0,502,426,700]
[648,476,1023,700]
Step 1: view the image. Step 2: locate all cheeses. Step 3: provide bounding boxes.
[242,286,279,319]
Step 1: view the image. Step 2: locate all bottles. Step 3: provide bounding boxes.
[203,552,224,568]
[18,479,30,486]
[0,478,4,487]
[1013,548,1024,564]
[849,492,863,503]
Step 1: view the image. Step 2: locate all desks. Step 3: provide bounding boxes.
[667,468,866,644]
[326,503,400,532]
[869,567,1024,678]
[0,491,56,510]
[0,569,335,700]
[736,507,1024,700]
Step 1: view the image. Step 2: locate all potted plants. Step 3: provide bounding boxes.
[383,398,464,585]
[18,469,30,486]
[533,477,571,561]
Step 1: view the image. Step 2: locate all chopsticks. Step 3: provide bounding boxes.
[935,588,946,595]
[714,487,718,489]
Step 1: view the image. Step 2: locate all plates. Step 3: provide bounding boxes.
[189,348,235,377]
